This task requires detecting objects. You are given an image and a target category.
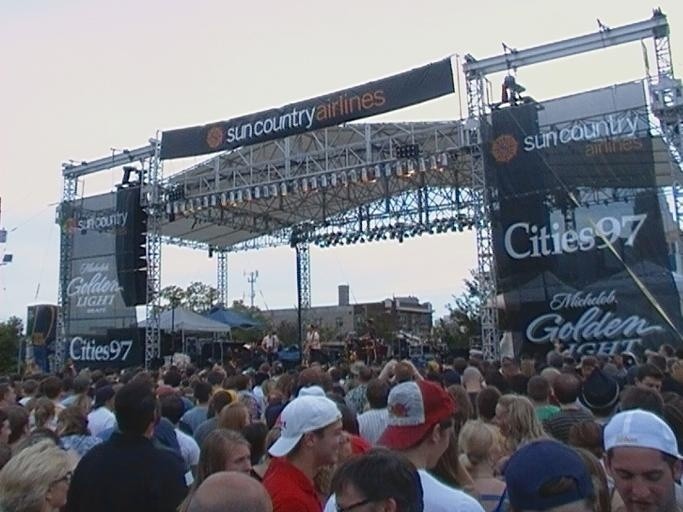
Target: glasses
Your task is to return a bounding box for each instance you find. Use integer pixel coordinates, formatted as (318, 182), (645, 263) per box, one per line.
(52, 471), (72, 485)
(335, 499), (374, 512)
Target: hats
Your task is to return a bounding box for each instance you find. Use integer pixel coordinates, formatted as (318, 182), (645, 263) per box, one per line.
(604, 409), (683, 461)
(376, 381), (456, 450)
(505, 440), (594, 511)
(93, 385), (114, 408)
(268, 395), (342, 458)
(579, 373), (621, 414)
(73, 375), (91, 387)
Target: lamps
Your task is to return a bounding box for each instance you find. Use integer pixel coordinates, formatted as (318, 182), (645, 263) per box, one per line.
(351, 170), (357, 183)
(430, 155), (437, 170)
(375, 164), (383, 178)
(418, 158), (425, 172)
(210, 195), (216, 207)
(396, 161), (403, 176)
(302, 178), (307, 193)
(362, 168), (367, 180)
(174, 201), (179, 213)
(203, 197), (209, 209)
(237, 190), (242, 200)
(441, 153), (448, 166)
(255, 187), (260, 198)
(384, 163), (391, 176)
(312, 176), (317, 189)
(281, 183), (287, 196)
(246, 188), (251, 200)
(195, 198), (201, 211)
(230, 192), (234, 202)
(342, 171), (347, 185)
(408, 159), (416, 173)
(270, 184), (277, 196)
(221, 194), (226, 203)
(183, 199), (195, 219)
(263, 186), (268, 197)
(292, 181), (298, 194)
(307, 214), (475, 248)
(321, 175), (327, 188)
(167, 204), (171, 213)
(331, 173), (337, 188)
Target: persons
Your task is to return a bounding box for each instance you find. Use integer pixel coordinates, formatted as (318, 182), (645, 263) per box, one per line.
(2, 325), (682, 512)
